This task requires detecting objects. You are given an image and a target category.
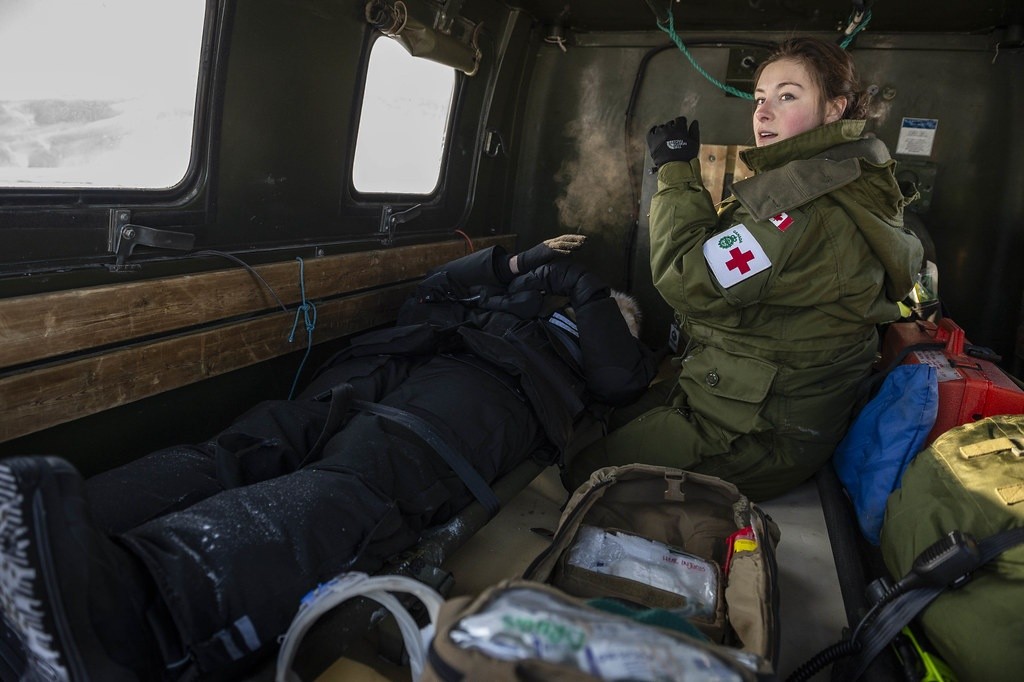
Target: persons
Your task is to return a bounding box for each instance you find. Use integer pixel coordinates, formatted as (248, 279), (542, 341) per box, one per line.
(558, 36), (925, 506)
(0, 233), (644, 682)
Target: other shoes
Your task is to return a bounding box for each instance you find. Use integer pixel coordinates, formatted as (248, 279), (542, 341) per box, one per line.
(1, 456), (153, 682)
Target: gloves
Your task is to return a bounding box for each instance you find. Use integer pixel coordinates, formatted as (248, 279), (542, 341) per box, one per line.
(516, 234), (587, 273)
(509, 263), (611, 306)
(646, 116), (700, 166)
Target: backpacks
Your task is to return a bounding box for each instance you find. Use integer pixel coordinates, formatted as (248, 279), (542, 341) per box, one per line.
(427, 461), (781, 682)
